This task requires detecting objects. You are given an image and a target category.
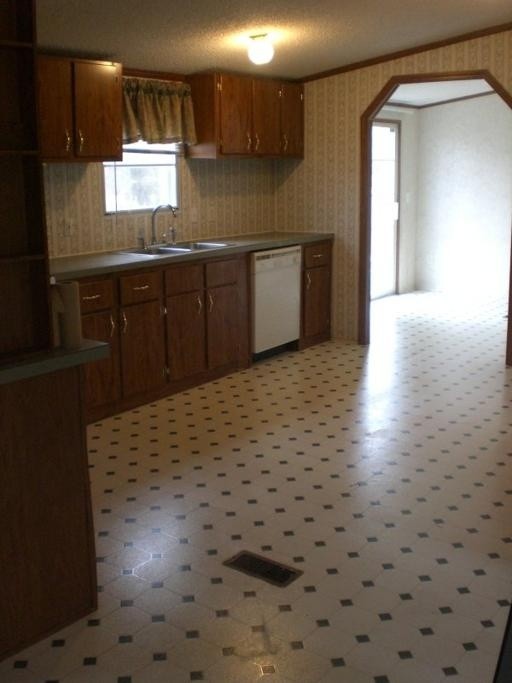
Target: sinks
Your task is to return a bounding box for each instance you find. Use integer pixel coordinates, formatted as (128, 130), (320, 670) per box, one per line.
(162, 242), (227, 250)
(126, 248), (186, 256)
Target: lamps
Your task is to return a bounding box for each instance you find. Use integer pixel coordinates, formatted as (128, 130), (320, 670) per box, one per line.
(247, 34), (274, 64)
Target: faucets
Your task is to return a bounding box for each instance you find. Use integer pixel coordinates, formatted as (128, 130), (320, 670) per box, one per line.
(151, 203), (176, 244)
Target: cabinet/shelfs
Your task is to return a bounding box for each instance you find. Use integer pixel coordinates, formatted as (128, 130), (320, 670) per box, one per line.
(185, 72), (281, 160)
(33, 53), (123, 161)
(72, 256), (169, 424)
(1, 1), (111, 661)
(164, 244), (250, 395)
(281, 81), (304, 158)
(287, 234), (331, 350)
